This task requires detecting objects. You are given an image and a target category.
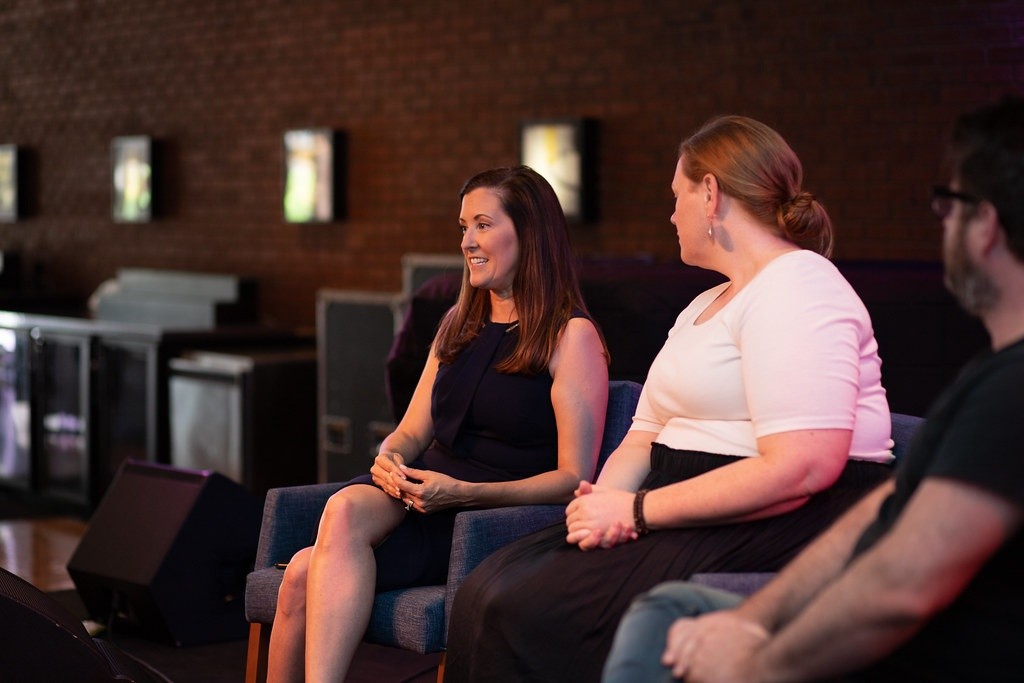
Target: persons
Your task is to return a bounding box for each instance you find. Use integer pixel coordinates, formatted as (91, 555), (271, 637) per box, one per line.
(663, 110), (1024, 683)
(266, 168), (608, 683)
(444, 118), (894, 682)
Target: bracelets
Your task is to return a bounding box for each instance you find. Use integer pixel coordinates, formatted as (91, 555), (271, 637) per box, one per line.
(633, 490), (648, 536)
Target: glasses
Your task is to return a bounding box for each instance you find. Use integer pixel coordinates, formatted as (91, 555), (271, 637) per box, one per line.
(927, 185), (980, 216)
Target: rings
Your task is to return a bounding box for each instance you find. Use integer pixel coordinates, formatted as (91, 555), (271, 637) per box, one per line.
(405, 500), (413, 511)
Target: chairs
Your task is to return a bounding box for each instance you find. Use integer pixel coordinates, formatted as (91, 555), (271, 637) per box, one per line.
(685, 410), (922, 597)
(244, 380), (644, 683)
